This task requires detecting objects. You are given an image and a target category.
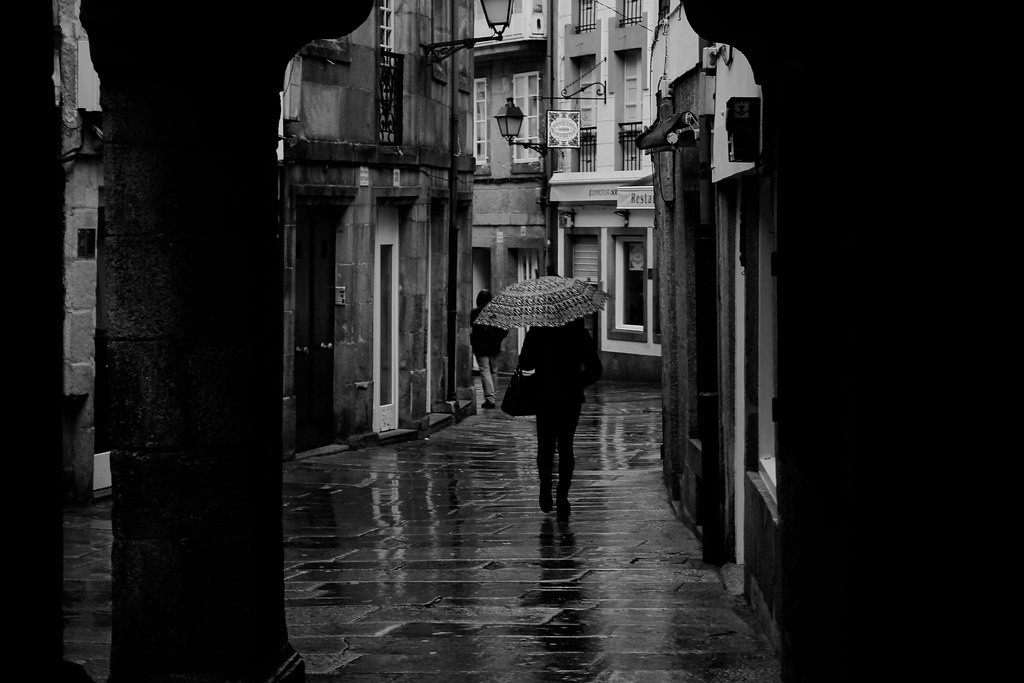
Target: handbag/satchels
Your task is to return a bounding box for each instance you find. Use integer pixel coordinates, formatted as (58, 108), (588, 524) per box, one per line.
(502, 365), (547, 417)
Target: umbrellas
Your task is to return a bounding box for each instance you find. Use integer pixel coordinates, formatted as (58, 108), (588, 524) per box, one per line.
(473, 276), (611, 331)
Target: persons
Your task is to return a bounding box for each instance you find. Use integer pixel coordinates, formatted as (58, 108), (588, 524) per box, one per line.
(518, 317), (604, 513)
(470, 289), (509, 409)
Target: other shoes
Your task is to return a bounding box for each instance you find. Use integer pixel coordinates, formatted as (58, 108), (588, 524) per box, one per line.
(482, 401), (496, 409)
(539, 475), (553, 513)
(556, 487), (571, 511)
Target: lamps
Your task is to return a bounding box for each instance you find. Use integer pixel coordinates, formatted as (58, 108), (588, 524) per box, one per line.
(419, 0), (514, 74)
(494, 96), (548, 156)
